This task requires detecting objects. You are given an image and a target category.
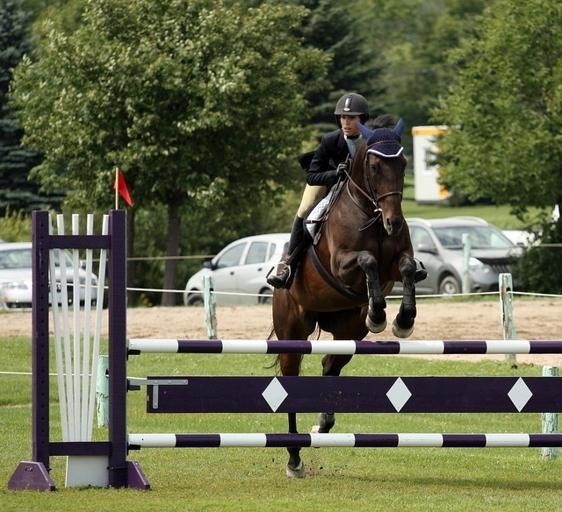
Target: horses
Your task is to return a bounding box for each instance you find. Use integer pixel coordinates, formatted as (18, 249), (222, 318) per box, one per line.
(262, 113), (418, 478)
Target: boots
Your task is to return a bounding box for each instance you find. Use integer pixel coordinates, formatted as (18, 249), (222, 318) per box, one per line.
(266, 255), (297, 290)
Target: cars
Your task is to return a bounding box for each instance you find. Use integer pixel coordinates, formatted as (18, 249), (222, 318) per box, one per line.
(184, 233), (290, 306)
(389, 219), (525, 300)
(1, 241), (101, 312)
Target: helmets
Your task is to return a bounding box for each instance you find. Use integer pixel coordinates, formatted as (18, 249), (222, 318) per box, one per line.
(334, 92), (370, 132)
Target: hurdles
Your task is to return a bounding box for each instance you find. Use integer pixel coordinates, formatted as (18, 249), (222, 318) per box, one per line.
(9, 210), (562, 494)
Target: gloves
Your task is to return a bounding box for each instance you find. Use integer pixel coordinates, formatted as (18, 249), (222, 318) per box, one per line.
(335, 162), (348, 177)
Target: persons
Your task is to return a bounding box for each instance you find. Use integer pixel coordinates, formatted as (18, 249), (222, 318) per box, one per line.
(267, 92), (428, 290)
